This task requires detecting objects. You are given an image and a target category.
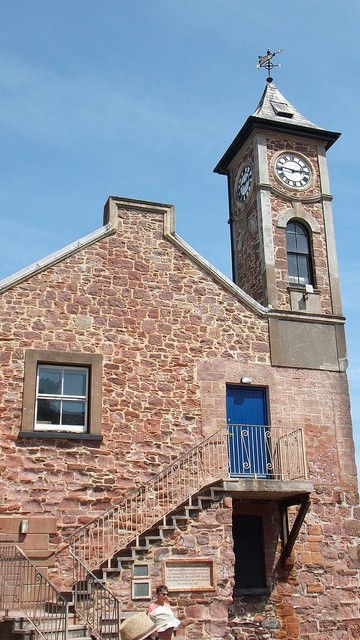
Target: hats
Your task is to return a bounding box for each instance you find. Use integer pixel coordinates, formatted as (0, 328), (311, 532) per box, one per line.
(150, 605), (180, 632)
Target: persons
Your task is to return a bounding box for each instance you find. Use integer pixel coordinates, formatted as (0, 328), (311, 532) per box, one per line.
(148, 586), (171, 611)
(149, 606), (181, 640)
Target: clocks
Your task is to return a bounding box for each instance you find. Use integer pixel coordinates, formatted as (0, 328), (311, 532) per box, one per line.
(234, 160), (254, 208)
(272, 150), (317, 192)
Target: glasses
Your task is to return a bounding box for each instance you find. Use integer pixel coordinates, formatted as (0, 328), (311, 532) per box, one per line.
(158, 593), (168, 595)
